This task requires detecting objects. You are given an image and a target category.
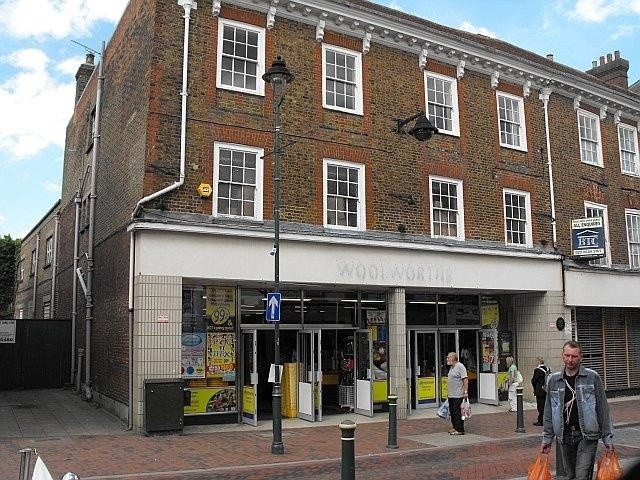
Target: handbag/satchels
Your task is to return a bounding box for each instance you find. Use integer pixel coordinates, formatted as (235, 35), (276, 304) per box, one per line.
(517, 370), (524, 384)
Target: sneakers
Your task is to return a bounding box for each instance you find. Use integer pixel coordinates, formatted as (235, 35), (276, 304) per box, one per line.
(448, 429), (456, 433)
(450, 431), (466, 436)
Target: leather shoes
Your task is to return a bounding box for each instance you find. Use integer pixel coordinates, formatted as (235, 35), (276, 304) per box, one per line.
(533, 422), (542, 426)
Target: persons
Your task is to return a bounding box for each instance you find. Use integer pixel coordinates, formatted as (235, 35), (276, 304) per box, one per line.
(445, 351), (469, 436)
(540, 341), (613, 480)
(506, 356), (523, 412)
(531, 355), (550, 425)
(373, 345), (385, 368)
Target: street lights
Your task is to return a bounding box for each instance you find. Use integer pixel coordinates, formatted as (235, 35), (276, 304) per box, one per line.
(259, 52), (296, 455)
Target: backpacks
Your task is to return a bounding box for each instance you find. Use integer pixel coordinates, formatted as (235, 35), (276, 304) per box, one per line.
(538, 366), (552, 390)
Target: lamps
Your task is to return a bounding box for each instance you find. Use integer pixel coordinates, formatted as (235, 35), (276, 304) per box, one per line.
(393, 109), (440, 141)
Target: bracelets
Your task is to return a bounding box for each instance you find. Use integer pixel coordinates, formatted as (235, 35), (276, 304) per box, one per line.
(464, 391), (468, 394)
